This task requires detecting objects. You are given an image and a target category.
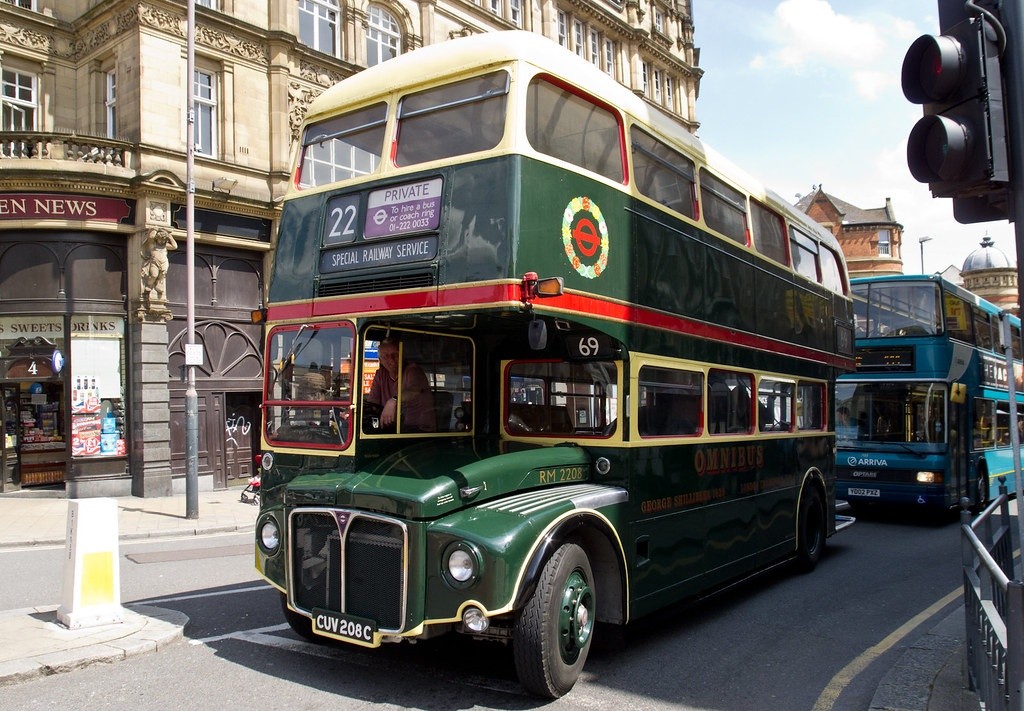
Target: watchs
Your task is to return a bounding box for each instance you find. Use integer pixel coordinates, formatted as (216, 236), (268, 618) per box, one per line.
(391, 395), (398, 402)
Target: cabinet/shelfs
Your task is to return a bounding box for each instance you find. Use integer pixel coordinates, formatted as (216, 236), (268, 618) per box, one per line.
(0, 382), (64, 488)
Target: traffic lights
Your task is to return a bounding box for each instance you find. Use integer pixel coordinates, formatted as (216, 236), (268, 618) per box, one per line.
(900, 12), (1011, 208)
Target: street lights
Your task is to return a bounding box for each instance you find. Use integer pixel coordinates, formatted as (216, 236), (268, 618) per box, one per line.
(919, 235), (932, 272)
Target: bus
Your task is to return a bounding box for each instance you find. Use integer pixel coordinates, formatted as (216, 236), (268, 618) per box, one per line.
(835, 274), (1024, 528)
(246, 29), (856, 698)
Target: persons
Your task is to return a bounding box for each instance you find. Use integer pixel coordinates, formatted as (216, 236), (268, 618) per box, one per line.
(362, 342), (444, 433)
(836, 406), (869, 442)
(145, 224), (179, 296)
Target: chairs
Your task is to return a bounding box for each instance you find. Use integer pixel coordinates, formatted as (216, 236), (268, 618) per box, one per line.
(430, 334), (1023, 448)
